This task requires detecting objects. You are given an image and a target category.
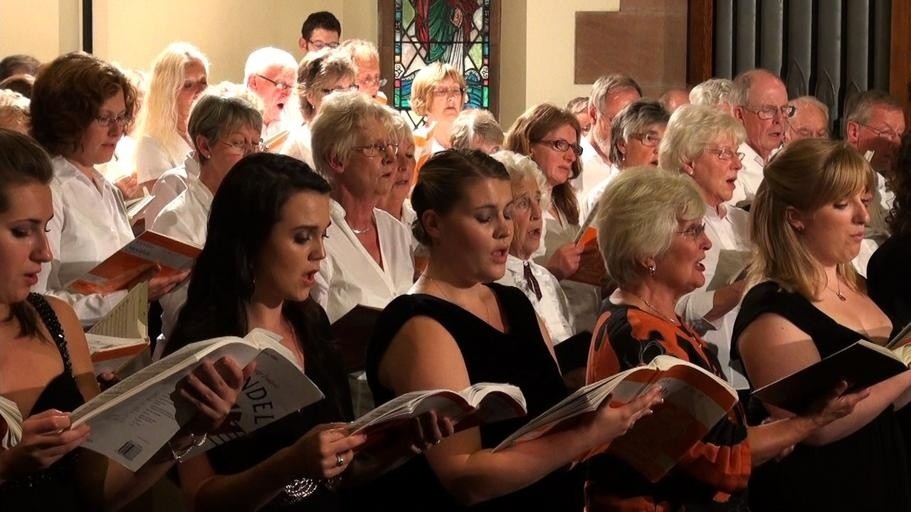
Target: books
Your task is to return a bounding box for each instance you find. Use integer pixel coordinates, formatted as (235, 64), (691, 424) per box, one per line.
(0, 396), (25, 451)
(885, 313), (911, 350)
(568, 200), (618, 290)
(491, 353), (739, 486)
(749, 321), (911, 436)
(376, 90), (388, 104)
(84, 279), (151, 380)
(727, 250), (764, 286)
(330, 303), (384, 371)
(336, 382), (527, 450)
(262, 130), (290, 153)
(125, 195), (156, 220)
(862, 149), (876, 167)
(412, 120), (437, 186)
(63, 229), (206, 295)
(55, 327), (325, 474)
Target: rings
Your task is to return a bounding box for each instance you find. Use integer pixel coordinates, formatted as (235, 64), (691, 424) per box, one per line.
(430, 438), (442, 446)
(337, 453), (343, 467)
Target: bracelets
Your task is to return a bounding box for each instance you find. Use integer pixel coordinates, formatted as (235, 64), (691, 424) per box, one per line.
(189, 428), (208, 448)
(167, 439), (194, 465)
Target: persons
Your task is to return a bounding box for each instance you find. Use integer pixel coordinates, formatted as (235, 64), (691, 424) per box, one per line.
(281, 48), (360, 174)
(839, 90), (907, 278)
(0, 53), (42, 137)
(609, 99), (669, 172)
(727, 70), (789, 208)
(365, 147), (664, 511)
(582, 163), (871, 512)
(112, 41), (210, 185)
(567, 97), (593, 137)
(18, 50), (193, 380)
(731, 140), (911, 512)
(448, 108), (506, 157)
(243, 47), (307, 155)
(298, 11), (342, 58)
(377, 104), (429, 263)
(688, 77), (736, 116)
(489, 150), (577, 348)
(503, 103), (607, 336)
(656, 104), (768, 390)
(149, 82), (265, 251)
(569, 72), (645, 230)
(787, 95), (830, 146)
(658, 87), (691, 115)
(408, 61), (469, 200)
(866, 132), (911, 341)
(0, 127), (257, 512)
(341, 38), (388, 103)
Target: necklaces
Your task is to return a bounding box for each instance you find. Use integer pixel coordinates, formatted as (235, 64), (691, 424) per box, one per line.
(818, 275), (847, 303)
(346, 206), (375, 235)
(621, 286), (696, 341)
(427, 260), (490, 326)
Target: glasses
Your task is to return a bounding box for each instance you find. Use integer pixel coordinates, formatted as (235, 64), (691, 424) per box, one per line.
(220, 139), (263, 153)
(534, 139), (583, 156)
(738, 103), (796, 119)
(94, 113), (128, 126)
(858, 122), (904, 143)
(357, 76), (388, 87)
(305, 39), (339, 50)
(352, 143), (400, 157)
(256, 74), (294, 92)
(674, 223), (707, 240)
(706, 148), (745, 161)
(630, 131), (662, 146)
(319, 84), (359, 94)
